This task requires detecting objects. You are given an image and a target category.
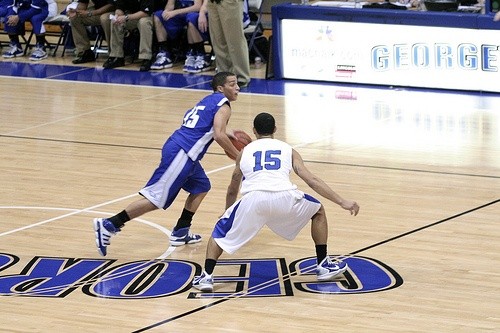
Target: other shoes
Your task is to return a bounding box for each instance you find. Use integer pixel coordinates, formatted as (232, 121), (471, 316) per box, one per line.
(71, 49), (97, 64)
(139, 59), (152, 72)
(237, 80), (248, 89)
(103, 56), (125, 69)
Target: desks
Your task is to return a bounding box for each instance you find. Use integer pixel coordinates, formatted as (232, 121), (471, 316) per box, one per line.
(272, 4), (500, 97)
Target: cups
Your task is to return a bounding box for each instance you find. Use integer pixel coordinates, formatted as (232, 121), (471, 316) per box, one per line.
(254, 57), (261, 69)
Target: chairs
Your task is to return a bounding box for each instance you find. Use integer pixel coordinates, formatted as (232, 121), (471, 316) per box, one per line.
(0, 0), (266, 65)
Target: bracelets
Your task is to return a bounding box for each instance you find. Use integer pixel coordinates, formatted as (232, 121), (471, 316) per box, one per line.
(124, 14), (128, 22)
(88, 10), (92, 17)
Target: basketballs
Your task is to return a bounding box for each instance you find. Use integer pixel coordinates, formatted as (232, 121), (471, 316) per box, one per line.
(224, 131), (252, 160)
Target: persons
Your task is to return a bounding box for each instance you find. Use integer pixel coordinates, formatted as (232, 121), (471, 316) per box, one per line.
(0, 0), (264, 90)
(192, 113), (360, 291)
(94, 72), (240, 257)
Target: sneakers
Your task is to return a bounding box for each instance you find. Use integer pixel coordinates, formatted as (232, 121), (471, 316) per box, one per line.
(2, 43), (24, 58)
(315, 255), (348, 282)
(191, 268), (214, 292)
(183, 51), (212, 74)
(29, 42), (48, 61)
(93, 217), (122, 257)
(150, 51), (174, 70)
(169, 226), (202, 246)
